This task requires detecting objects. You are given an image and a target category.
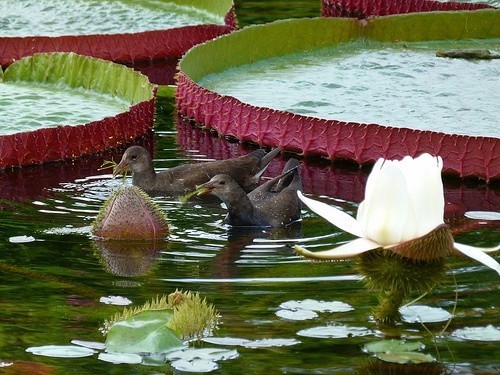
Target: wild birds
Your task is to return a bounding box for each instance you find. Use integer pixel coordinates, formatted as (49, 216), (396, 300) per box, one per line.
(196, 157), (304, 228)
(111, 144), (282, 192)
